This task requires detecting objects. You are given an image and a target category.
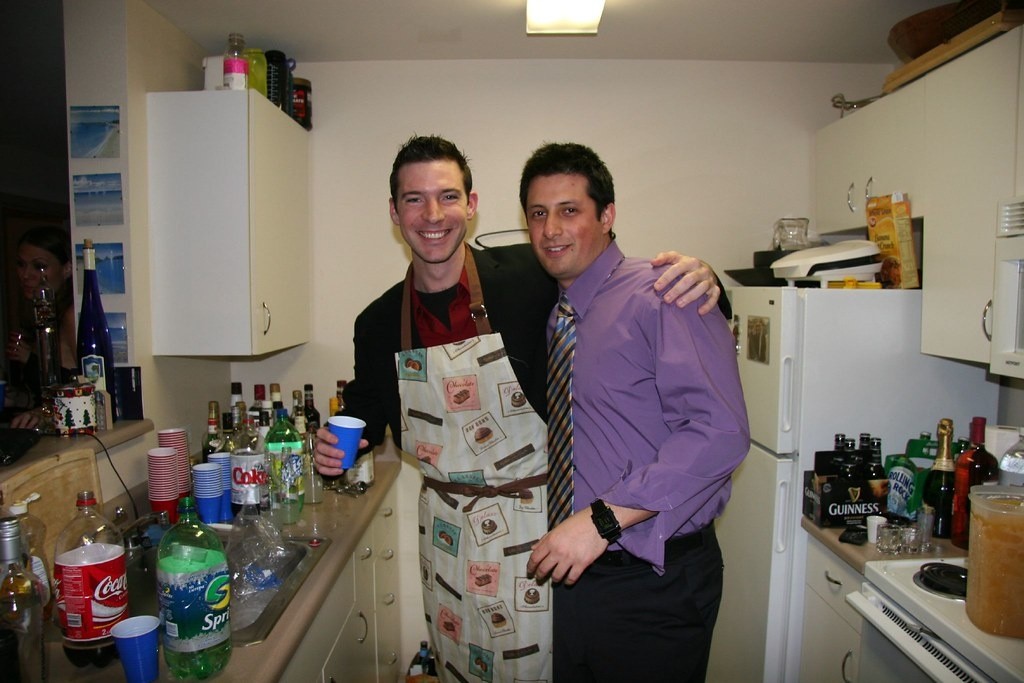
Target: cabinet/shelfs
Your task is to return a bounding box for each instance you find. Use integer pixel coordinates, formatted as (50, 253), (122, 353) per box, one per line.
(275, 469), (407, 683)
(797, 530), (875, 682)
(812, 89), (924, 235)
(919, 24), (1024, 381)
(142, 92), (316, 359)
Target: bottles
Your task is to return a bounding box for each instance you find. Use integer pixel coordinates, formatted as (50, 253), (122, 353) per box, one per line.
(0, 490), (128, 683)
(223, 33), (313, 131)
(773, 218), (812, 251)
(829, 434), (888, 495)
(202, 420), (222, 464)
(920, 417), (1024, 551)
(214, 413), (241, 452)
(32, 239), (116, 434)
(208, 401), (219, 419)
(155, 496), (232, 683)
(232, 380), (375, 527)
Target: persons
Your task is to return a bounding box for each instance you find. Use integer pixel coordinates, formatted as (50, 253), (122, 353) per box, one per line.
(6, 225), (79, 434)
(312, 132), (734, 683)
(517, 138), (751, 683)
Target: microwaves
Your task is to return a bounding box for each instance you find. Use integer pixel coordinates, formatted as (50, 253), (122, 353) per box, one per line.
(990, 195), (1024, 381)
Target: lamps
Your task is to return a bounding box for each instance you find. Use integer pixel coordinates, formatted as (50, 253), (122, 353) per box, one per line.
(525, 0), (607, 35)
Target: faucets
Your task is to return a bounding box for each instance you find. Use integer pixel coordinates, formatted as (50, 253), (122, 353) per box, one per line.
(76, 506), (170, 546)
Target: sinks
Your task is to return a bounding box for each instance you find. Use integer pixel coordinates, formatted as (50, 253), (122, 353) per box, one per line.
(116, 510), (333, 648)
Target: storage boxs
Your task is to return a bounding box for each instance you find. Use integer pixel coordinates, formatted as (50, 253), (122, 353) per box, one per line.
(869, 189), (919, 292)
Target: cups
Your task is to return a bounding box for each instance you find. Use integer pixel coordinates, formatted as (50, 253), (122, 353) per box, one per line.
(109, 613), (161, 683)
(147, 428), (231, 524)
(51, 376), (97, 437)
(0, 380), (6, 412)
(327, 416), (366, 469)
(866, 507), (919, 554)
(917, 506), (936, 552)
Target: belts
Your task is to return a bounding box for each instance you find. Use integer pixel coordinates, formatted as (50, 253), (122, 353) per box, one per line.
(594, 522), (716, 567)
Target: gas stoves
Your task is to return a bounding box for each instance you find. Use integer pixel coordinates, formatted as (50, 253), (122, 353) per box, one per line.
(864, 557), (1024, 683)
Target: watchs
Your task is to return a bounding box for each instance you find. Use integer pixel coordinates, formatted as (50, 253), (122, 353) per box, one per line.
(588, 498), (623, 544)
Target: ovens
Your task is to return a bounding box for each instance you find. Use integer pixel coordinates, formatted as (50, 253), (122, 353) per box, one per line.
(845, 582), (999, 683)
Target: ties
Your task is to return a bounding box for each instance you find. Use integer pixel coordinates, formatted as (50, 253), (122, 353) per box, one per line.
(547, 258), (624, 531)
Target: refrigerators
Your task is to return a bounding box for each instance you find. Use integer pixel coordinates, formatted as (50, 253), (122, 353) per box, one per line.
(705, 286), (1000, 683)
